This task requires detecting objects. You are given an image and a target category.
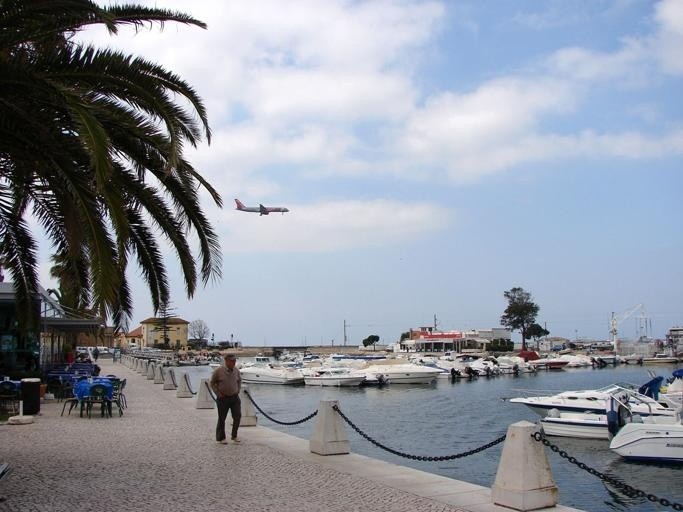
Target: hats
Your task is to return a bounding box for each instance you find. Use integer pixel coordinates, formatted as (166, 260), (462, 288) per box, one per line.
(224, 354), (239, 360)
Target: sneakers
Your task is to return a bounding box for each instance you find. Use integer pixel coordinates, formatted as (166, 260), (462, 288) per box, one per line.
(231, 436), (241, 442)
(220, 439), (228, 444)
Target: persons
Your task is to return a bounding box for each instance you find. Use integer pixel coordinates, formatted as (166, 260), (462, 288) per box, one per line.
(208, 350), (243, 445)
(91, 345), (98, 361)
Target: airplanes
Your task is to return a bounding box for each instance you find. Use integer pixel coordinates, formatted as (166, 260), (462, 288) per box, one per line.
(233, 199), (289, 216)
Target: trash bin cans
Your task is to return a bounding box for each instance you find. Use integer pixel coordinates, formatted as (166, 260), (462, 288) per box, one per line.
(20, 378), (41, 415)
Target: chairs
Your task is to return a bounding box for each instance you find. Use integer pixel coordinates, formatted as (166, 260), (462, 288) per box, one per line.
(0, 359), (127, 419)
